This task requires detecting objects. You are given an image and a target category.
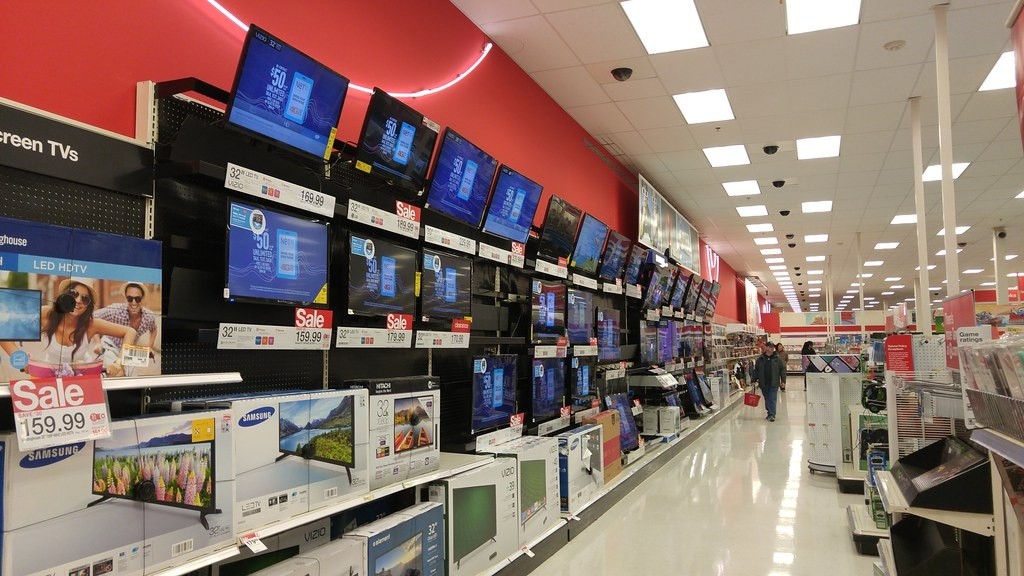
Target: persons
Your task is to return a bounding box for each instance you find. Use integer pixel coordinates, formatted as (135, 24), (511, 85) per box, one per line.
(0, 277), (136, 378)
(90, 282), (157, 376)
(802, 341), (816, 391)
(773, 343), (789, 391)
(751, 341), (786, 421)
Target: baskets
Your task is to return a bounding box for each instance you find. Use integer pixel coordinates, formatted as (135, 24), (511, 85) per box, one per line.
(745, 393), (761, 407)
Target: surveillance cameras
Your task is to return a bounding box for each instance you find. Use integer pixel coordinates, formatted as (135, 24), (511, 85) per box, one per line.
(773, 180), (785, 188)
(611, 68), (633, 82)
(997, 230), (1007, 238)
(780, 211), (790, 216)
(786, 235), (807, 310)
(763, 146), (778, 155)
(958, 243), (967, 247)
(935, 293), (939, 295)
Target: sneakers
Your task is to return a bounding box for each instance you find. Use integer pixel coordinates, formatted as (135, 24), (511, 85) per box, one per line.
(768, 416), (775, 421)
(766, 412), (768, 419)
(780, 386), (785, 392)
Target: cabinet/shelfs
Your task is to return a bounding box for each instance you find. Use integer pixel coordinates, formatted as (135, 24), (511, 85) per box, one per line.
(872, 469), (994, 576)
(781, 343), (830, 373)
(153, 451), (511, 576)
(725, 323), (769, 393)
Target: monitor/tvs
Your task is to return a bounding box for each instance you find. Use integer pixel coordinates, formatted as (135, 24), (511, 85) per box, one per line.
(226, 21), (722, 453)
(393, 395), (434, 454)
(452, 484), (496, 571)
(374, 530), (425, 576)
(520, 459), (547, 532)
(88, 417), (222, 530)
(276, 395), (355, 485)
(0, 287), (42, 348)
(581, 428), (600, 484)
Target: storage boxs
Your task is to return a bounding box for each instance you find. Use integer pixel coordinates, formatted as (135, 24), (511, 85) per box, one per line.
(0, 368), (729, 576)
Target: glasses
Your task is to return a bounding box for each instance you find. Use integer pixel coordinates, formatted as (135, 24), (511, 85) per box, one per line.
(642, 188), (649, 198)
(127, 295), (141, 303)
(652, 197), (659, 204)
(69, 288), (90, 305)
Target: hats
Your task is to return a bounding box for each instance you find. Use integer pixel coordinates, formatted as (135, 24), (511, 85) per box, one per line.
(120, 282), (149, 301)
(58, 277), (98, 305)
(766, 342), (777, 347)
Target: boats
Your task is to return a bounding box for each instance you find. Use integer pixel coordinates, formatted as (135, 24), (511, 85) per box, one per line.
(418, 427), (430, 447)
(395, 426), (415, 452)
(394, 430), (405, 451)
